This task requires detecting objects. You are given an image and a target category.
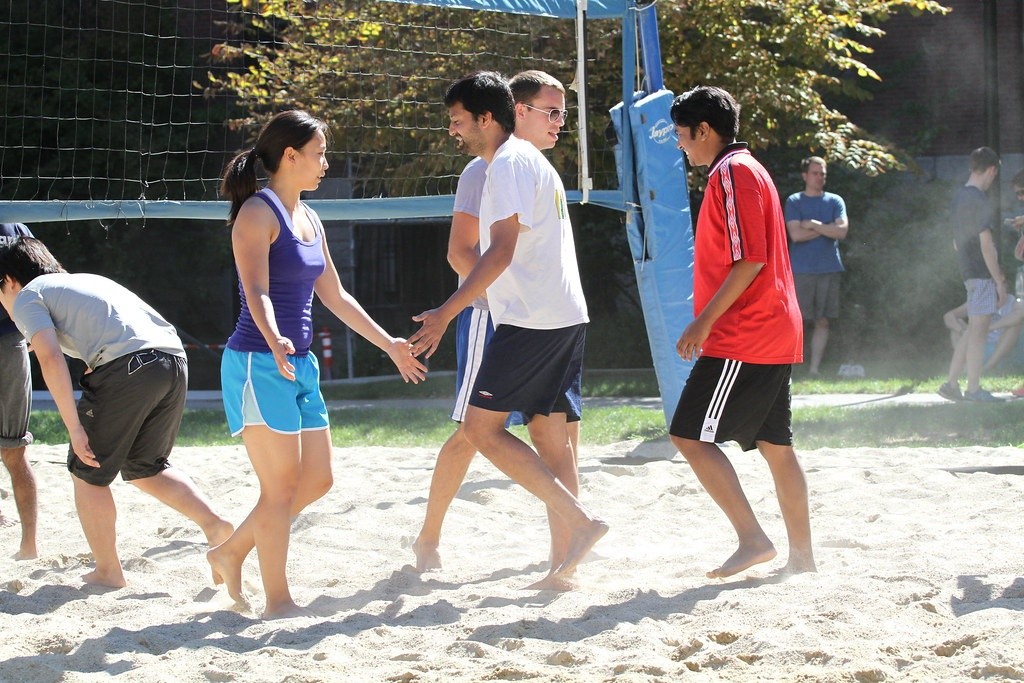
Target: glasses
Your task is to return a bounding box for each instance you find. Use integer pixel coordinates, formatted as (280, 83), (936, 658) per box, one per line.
(519, 102), (568, 124)
(1015, 189), (1024, 196)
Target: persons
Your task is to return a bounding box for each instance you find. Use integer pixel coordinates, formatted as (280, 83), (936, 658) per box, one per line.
(415, 71), (586, 570)
(207, 110), (427, 622)
(937, 147), (1024, 402)
(0, 223), (38, 561)
(785, 156), (848, 380)
(669, 86), (819, 577)
(0, 237), (234, 587)
(404, 71), (608, 580)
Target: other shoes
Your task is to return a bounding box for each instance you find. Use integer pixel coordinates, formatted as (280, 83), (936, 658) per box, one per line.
(1011, 381), (1024, 396)
(935, 381), (964, 402)
(964, 388), (1005, 403)
(807, 370), (824, 380)
